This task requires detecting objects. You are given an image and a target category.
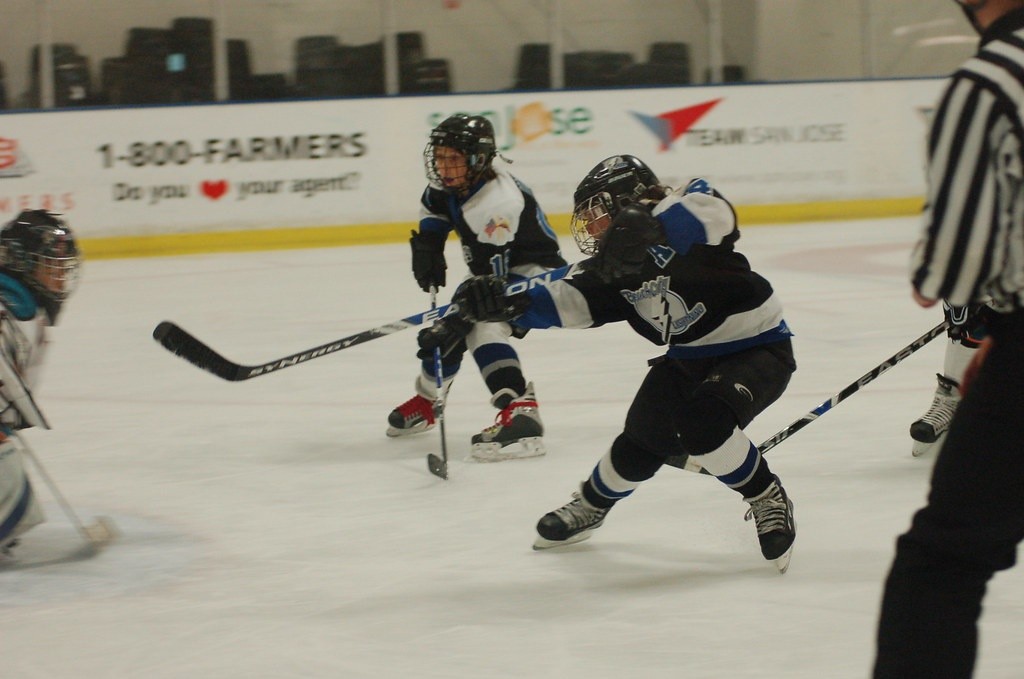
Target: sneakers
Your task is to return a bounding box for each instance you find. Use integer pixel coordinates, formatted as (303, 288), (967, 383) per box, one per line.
(743, 474), (796, 574)
(385, 376), (453, 437)
(470, 381), (547, 462)
(532, 480), (622, 550)
(909, 373), (962, 458)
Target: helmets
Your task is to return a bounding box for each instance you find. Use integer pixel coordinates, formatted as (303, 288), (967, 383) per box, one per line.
(423, 113), (496, 198)
(569, 154), (658, 255)
(0, 208), (79, 324)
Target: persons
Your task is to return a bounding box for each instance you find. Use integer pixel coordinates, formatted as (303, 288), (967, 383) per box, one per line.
(0, 209), (109, 573)
(387, 112), (567, 462)
(871, 0), (1024, 679)
(453, 153), (797, 573)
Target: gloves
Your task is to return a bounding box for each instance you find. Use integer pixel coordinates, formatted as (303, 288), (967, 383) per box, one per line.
(594, 203), (668, 284)
(450, 274), (524, 323)
(410, 229), (447, 292)
(943, 298), (993, 341)
(416, 313), (474, 361)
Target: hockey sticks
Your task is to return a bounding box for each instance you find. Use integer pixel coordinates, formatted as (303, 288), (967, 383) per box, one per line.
(152, 258), (595, 383)
(426, 277), (449, 481)
(650, 322), (949, 477)
(9, 427), (122, 547)
